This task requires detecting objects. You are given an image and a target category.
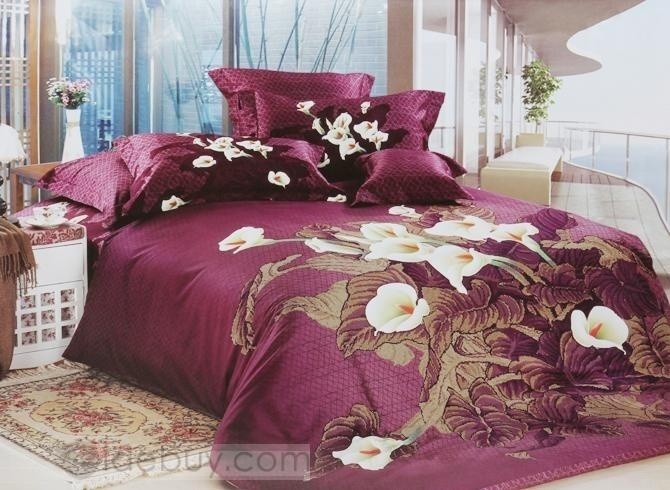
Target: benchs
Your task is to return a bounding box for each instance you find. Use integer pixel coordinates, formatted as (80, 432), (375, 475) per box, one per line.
(479, 144), (565, 207)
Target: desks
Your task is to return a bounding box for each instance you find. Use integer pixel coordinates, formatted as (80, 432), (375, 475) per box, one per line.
(9, 160), (61, 212)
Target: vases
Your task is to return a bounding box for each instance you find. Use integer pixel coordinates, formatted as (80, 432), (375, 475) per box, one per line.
(61, 109), (85, 167)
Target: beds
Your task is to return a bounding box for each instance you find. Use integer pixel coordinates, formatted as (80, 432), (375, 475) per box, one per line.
(7, 186), (670, 490)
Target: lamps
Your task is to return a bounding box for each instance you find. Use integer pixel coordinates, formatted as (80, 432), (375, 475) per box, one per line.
(0, 123), (27, 217)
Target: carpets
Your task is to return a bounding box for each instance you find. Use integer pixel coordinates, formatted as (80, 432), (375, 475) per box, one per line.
(2, 364), (220, 479)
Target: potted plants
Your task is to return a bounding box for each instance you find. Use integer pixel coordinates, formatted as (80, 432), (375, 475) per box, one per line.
(514, 59), (566, 146)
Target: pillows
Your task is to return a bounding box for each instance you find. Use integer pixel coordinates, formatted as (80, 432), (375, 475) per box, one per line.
(207, 68), (376, 139)
(352, 149), (476, 212)
(116, 129), (342, 220)
(35, 149), (133, 231)
(234, 83), (445, 183)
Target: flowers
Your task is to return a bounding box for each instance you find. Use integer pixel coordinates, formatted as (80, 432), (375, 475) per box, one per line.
(46, 75), (96, 110)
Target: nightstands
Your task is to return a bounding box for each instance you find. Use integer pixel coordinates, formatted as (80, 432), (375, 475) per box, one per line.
(9, 220), (88, 373)
